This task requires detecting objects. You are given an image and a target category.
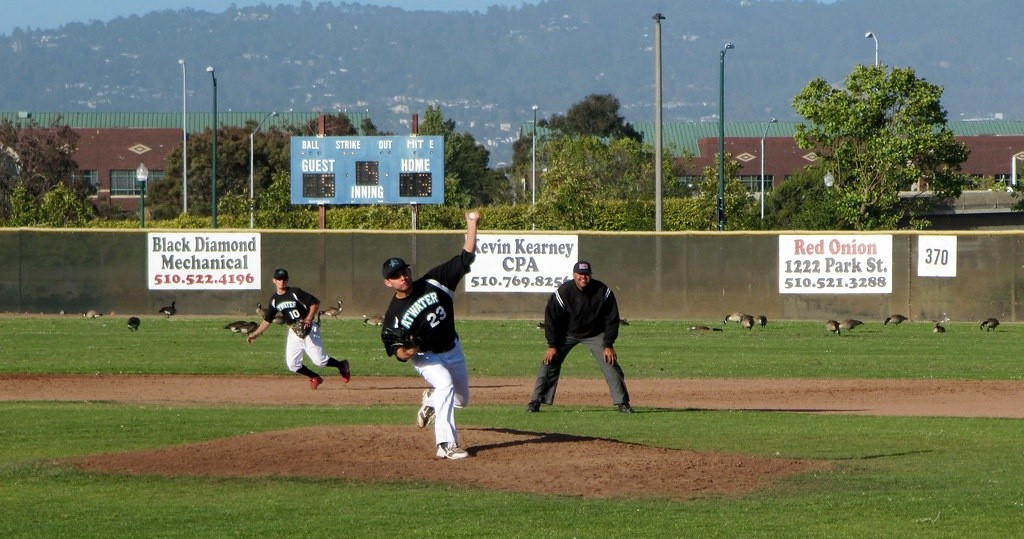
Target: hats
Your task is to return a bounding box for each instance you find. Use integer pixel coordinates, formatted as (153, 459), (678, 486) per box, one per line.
(382, 257), (410, 279)
(273, 269), (288, 279)
(573, 261), (592, 274)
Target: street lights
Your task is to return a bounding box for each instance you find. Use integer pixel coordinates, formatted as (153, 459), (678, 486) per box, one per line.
(865, 31), (880, 67)
(532, 105), (539, 230)
(651, 12), (666, 232)
(206, 66), (219, 228)
(136, 161), (148, 228)
(177, 59), (187, 214)
(249, 111), (280, 228)
(760, 116), (779, 219)
(718, 41), (735, 230)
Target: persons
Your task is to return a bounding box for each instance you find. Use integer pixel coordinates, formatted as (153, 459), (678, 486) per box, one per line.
(247, 268), (350, 390)
(529, 260), (633, 413)
(380, 206), (481, 459)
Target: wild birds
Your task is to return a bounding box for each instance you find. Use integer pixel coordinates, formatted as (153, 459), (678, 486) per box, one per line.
(323, 300), (343, 320)
(272, 317), (285, 326)
(537, 323), (545, 331)
(314, 311), (323, 326)
(256, 303), (268, 318)
(159, 301), (176, 318)
(127, 317), (140, 333)
(686, 325), (723, 332)
(620, 318), (629, 326)
(224, 321), (260, 336)
(362, 315), (385, 327)
(824, 313), (1001, 335)
(723, 312), (767, 330)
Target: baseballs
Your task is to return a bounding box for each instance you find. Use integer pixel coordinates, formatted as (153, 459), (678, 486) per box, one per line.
(469, 212), (476, 219)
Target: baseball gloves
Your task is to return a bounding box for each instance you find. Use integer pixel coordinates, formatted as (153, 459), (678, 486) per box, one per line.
(291, 321), (312, 338)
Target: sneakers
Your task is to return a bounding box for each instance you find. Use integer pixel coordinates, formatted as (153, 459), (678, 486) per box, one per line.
(416, 388), (436, 429)
(527, 400), (540, 412)
(437, 442), (469, 459)
(618, 403), (633, 414)
(338, 360), (350, 383)
(309, 375), (323, 390)
